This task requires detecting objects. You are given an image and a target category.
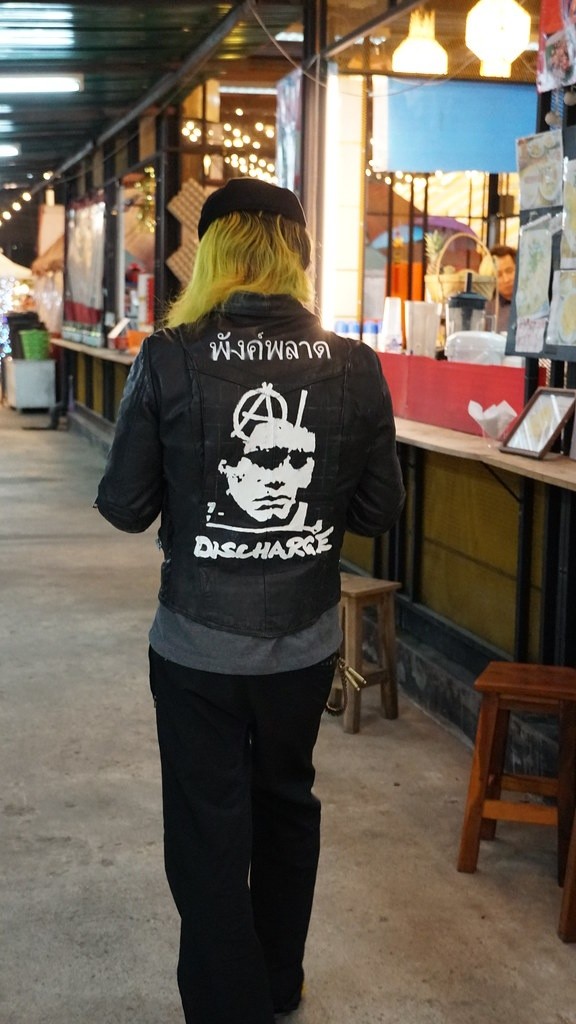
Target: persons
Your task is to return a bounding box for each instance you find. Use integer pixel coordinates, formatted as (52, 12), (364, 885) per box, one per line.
(485, 246), (516, 334)
(91, 178), (406, 1024)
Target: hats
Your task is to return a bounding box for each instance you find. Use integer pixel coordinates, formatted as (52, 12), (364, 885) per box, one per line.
(197, 178), (307, 241)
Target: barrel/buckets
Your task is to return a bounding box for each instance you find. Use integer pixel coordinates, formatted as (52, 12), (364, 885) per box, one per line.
(19, 330), (48, 361)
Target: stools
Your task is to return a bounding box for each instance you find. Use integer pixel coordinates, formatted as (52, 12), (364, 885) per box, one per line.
(327, 571), (403, 733)
(456, 661), (576, 944)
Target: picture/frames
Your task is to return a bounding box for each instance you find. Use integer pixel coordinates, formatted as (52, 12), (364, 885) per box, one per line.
(500, 385), (576, 460)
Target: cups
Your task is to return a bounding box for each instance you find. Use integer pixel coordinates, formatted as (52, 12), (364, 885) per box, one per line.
(333, 296), (443, 358)
